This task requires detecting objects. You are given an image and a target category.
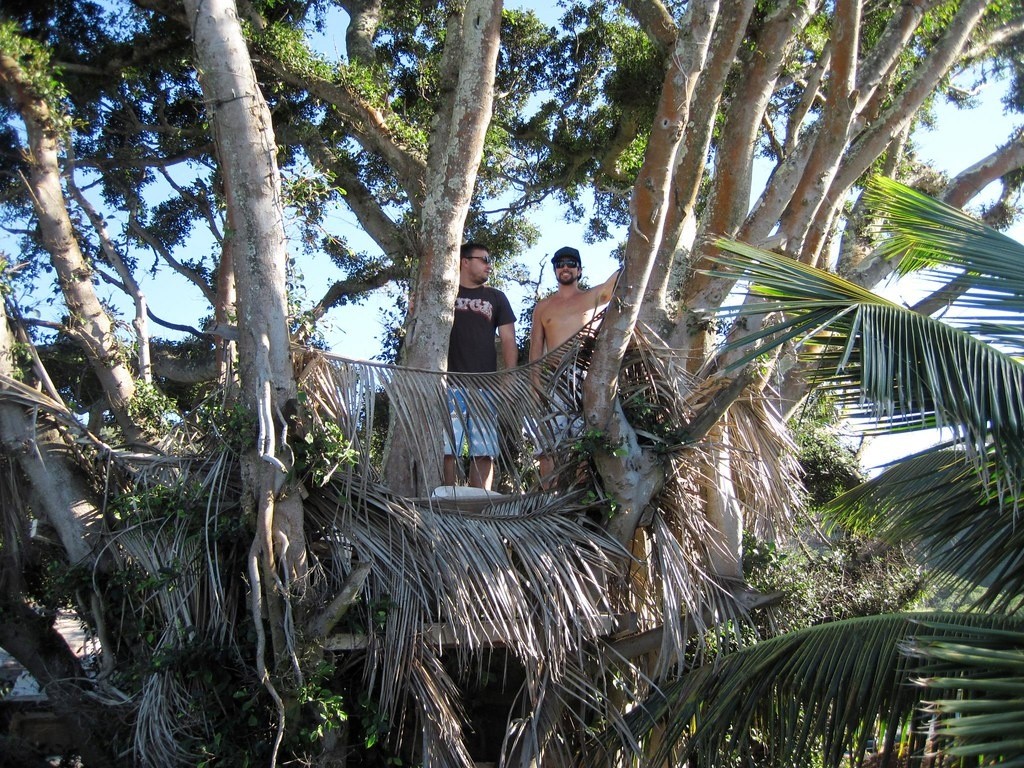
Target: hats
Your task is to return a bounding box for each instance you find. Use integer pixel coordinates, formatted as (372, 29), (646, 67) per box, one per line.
(551, 246), (581, 268)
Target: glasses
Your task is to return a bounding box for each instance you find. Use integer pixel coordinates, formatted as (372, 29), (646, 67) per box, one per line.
(465, 256), (491, 264)
(553, 259), (580, 268)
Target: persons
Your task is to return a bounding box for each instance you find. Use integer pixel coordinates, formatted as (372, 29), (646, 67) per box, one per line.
(406, 244), (518, 492)
(528, 246), (621, 491)
